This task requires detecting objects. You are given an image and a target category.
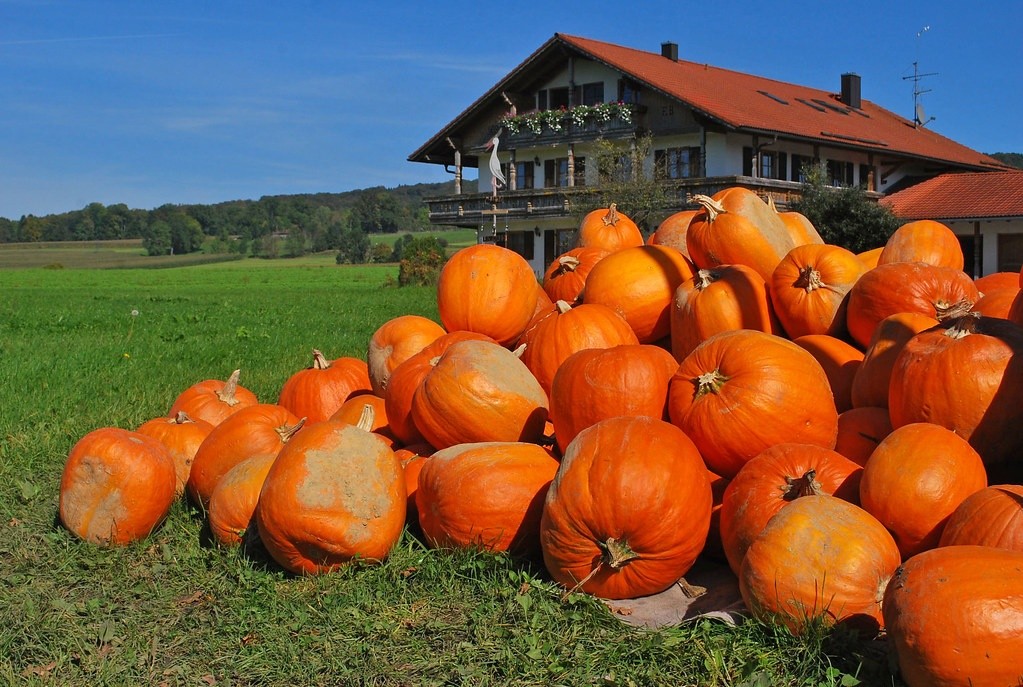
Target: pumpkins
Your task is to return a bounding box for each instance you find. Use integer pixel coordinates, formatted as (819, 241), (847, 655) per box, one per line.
(60, 188), (1023, 687)
